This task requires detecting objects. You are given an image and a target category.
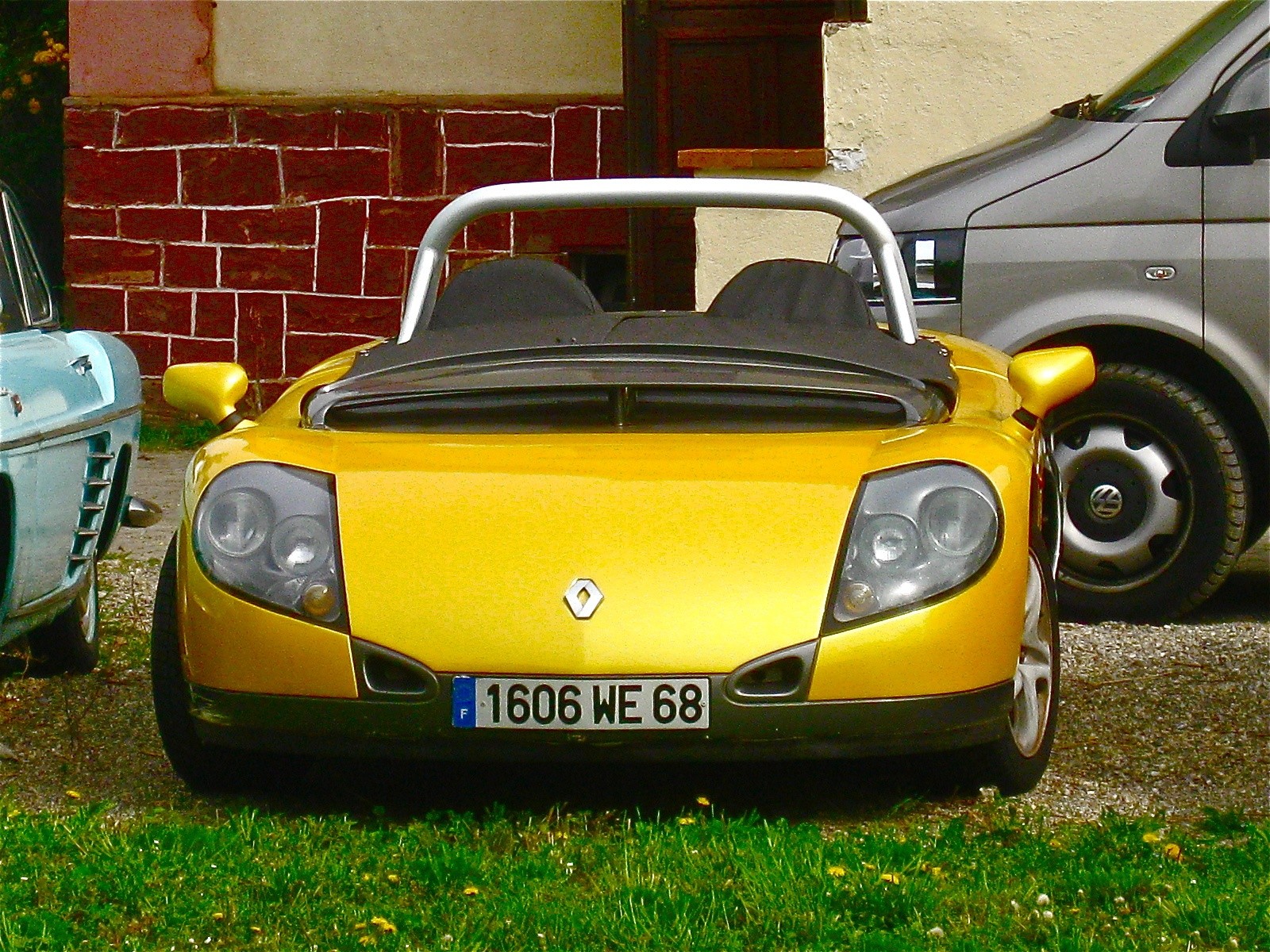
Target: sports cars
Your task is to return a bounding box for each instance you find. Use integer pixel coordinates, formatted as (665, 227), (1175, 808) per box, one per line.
(145, 171), (1102, 801)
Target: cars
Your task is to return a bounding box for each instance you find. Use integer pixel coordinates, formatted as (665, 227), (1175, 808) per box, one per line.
(0, 183), (141, 682)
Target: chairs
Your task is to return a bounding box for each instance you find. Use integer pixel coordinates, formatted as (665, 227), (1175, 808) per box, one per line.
(424, 256), (601, 329)
(705, 258), (879, 330)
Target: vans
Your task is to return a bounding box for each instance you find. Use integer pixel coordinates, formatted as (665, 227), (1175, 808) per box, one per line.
(830, 1), (1270, 628)
